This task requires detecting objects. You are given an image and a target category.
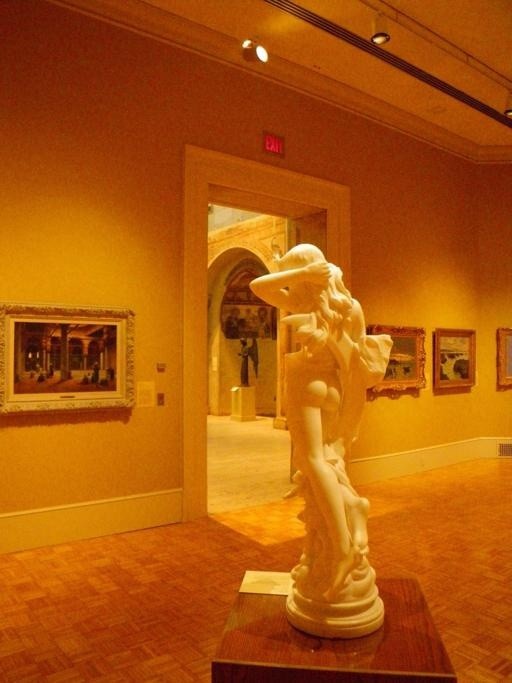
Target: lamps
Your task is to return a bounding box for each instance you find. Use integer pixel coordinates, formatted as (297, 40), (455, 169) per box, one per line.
(237, 36), (270, 68)
(370, 7), (392, 45)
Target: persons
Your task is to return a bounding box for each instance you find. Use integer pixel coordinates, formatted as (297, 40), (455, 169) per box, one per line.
(30, 360), (114, 386)
(225, 307), (271, 338)
(250, 242), (394, 595)
(238, 337), (249, 384)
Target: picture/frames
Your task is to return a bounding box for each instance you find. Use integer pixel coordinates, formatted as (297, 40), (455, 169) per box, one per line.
(495, 328), (511, 393)
(0, 304), (140, 420)
(367, 323), (478, 394)
